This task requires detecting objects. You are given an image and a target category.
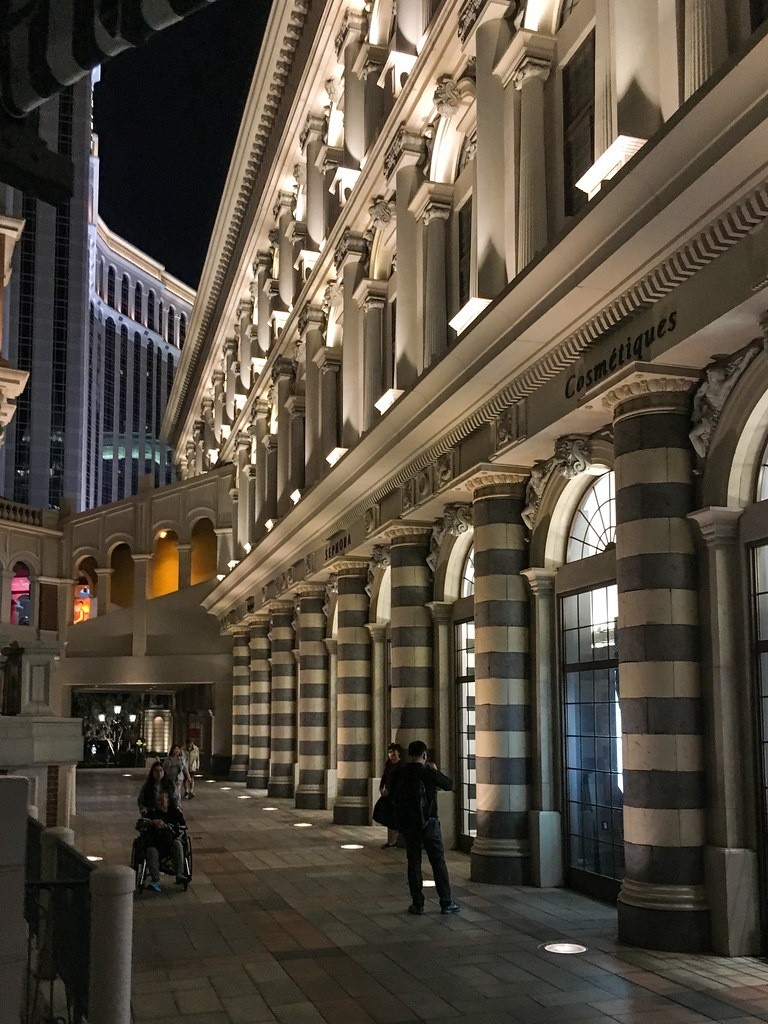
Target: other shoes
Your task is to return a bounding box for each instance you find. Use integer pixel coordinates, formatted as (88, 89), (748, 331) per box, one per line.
(184, 792), (189, 799)
(147, 882), (162, 892)
(407, 905), (425, 915)
(187, 792), (195, 800)
(383, 842), (398, 849)
(175, 876), (193, 885)
(440, 900), (462, 914)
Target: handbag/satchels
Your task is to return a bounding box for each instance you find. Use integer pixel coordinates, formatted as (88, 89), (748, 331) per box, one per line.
(372, 794), (393, 827)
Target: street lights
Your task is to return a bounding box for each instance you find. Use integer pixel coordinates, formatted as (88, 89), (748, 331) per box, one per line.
(98, 702), (137, 764)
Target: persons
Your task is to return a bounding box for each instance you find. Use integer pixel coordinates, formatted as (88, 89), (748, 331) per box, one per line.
(131, 761), (193, 893)
(163, 738), (199, 799)
(372, 741), (462, 915)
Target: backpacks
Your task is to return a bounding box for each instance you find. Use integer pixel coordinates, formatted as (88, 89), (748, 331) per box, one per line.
(392, 766), (431, 830)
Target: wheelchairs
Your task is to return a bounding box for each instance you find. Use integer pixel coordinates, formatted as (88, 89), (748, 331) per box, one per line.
(130, 832), (193, 894)
